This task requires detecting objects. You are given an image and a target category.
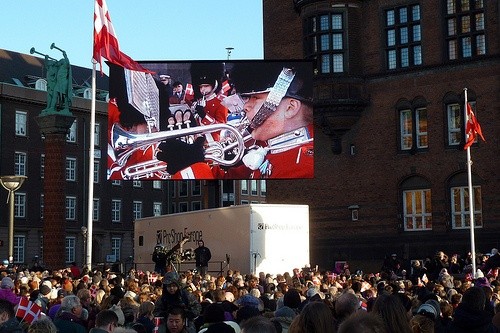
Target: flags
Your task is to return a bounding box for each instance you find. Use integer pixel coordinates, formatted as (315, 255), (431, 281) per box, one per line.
(16, 297), (34, 318)
(23, 303), (42, 323)
(221, 79), (231, 93)
(463, 102), (487, 151)
(90, 284), (99, 294)
(93, 0), (156, 78)
(465, 273), (472, 282)
(487, 270), (493, 276)
(149, 274), (158, 282)
(186, 83), (194, 95)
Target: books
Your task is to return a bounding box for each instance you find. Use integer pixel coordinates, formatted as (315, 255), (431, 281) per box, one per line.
(123, 66), (161, 132)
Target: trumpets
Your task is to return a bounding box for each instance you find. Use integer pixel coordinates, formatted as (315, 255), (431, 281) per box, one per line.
(110, 116), (258, 179)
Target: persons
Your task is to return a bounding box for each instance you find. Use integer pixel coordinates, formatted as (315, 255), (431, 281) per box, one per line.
(107, 60), (314, 179)
(43, 52), (71, 112)
(194, 240), (212, 275)
(152, 239), (167, 273)
(0, 248), (500, 333)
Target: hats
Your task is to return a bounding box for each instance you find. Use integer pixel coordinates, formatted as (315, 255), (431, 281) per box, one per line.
(482, 253), (491, 256)
(475, 269), (484, 280)
(124, 291), (136, 300)
(417, 304), (437, 320)
(490, 249), (498, 257)
(239, 60), (314, 102)
(173, 81), (181, 87)
(62, 279), (73, 291)
(1, 275), (14, 289)
(162, 272), (179, 284)
(306, 288), (318, 297)
(196, 71), (213, 84)
(39, 280), (52, 295)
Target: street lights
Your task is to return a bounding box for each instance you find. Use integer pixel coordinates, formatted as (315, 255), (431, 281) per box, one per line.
(0, 174), (29, 268)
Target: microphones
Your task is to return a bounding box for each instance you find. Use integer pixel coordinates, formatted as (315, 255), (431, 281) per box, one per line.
(226, 254), (230, 261)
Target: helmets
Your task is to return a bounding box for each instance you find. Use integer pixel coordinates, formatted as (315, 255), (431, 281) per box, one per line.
(233, 294), (264, 312)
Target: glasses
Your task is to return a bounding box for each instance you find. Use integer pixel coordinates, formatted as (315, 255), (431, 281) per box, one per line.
(174, 86), (181, 88)
